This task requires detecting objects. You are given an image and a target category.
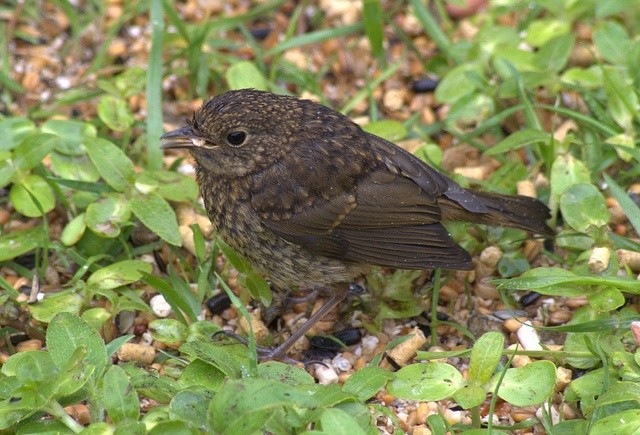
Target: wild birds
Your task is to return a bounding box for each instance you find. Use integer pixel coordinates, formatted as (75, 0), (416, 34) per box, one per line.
(158, 89), (557, 368)
